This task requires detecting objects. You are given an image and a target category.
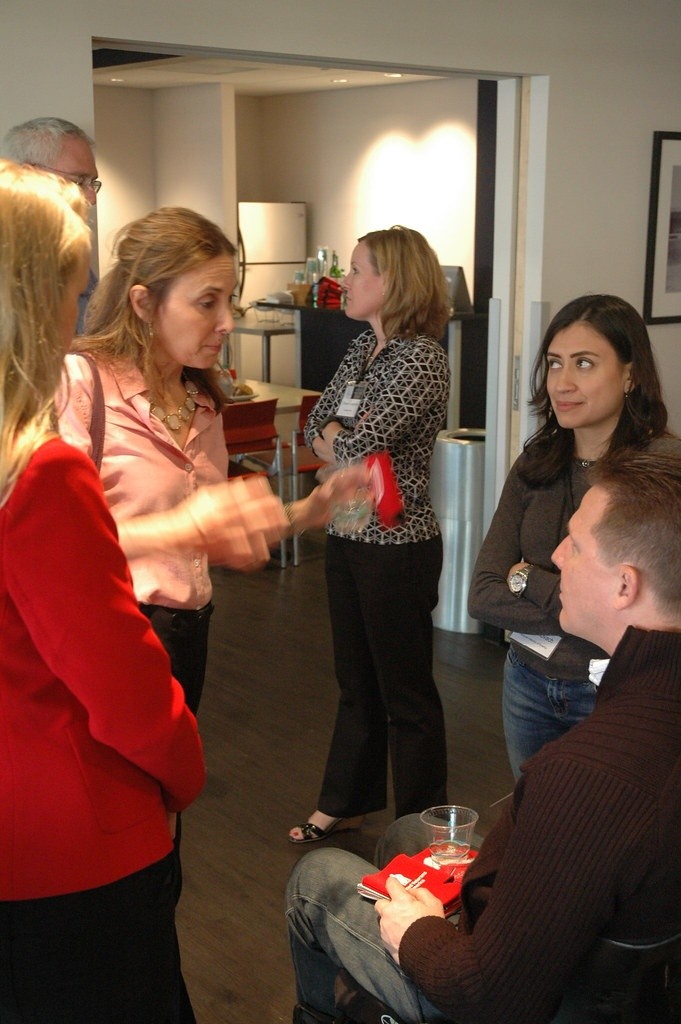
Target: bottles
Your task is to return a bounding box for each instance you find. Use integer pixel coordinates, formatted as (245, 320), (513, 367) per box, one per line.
(330, 250), (347, 285)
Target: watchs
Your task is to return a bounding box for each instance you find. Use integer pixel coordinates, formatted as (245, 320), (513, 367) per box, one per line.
(508, 565), (533, 599)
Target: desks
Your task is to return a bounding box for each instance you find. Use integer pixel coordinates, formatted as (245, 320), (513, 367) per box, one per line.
(234, 377), (323, 417)
(256, 301), (489, 429)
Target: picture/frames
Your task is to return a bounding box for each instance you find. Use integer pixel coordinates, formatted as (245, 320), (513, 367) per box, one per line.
(643, 131), (681, 325)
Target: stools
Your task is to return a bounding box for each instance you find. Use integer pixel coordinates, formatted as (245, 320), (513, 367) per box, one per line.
(232, 324), (295, 383)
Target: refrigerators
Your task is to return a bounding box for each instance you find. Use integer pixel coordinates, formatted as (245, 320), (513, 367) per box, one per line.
(227, 201), (306, 387)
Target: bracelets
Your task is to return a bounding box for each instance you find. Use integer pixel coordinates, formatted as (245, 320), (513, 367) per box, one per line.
(283, 503), (308, 538)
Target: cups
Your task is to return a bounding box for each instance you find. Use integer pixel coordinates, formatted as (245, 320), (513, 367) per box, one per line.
(315, 456), (371, 536)
(420, 805), (479, 864)
(292, 246), (329, 300)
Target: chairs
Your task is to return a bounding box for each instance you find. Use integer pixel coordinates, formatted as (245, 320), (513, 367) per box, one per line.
(222, 398), (287, 568)
(236, 395), (331, 565)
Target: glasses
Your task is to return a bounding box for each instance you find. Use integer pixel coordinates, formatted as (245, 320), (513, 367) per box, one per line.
(32, 162), (102, 194)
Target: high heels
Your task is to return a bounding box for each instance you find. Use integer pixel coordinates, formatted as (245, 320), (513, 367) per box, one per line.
(284, 810), (363, 843)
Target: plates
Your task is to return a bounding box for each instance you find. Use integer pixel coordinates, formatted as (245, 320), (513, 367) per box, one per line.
(230, 393), (259, 401)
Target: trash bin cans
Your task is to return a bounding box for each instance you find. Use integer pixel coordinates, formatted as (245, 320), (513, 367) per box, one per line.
(429, 429), (486, 637)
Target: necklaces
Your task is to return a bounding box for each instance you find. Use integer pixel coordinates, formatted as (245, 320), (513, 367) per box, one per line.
(137, 383), (201, 431)
(574, 422), (620, 468)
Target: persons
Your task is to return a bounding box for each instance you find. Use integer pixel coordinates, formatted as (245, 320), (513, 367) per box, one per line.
(468, 295), (681, 786)
(56, 207), (375, 907)
(290, 226), (451, 841)
(0, 164), (210, 1024)
(284, 448), (681, 1024)
(1, 117), (103, 336)
